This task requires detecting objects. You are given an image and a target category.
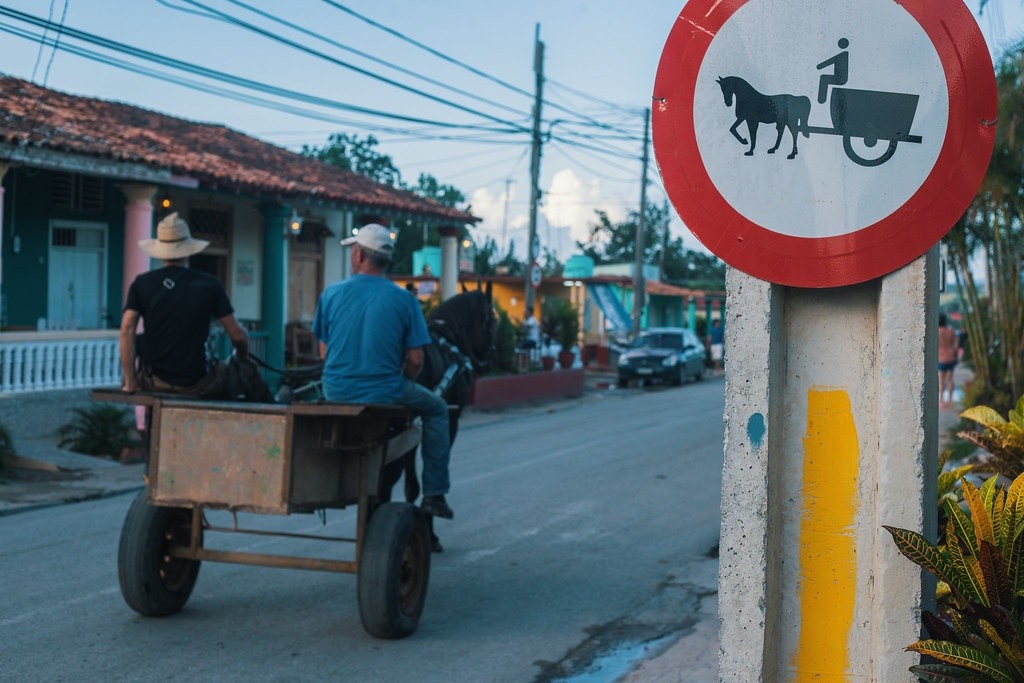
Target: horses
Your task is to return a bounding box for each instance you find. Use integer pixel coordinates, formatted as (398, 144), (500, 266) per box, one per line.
(367, 278), (499, 554)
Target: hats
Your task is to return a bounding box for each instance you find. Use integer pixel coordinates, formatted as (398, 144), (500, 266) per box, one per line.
(138, 211), (210, 260)
(340, 223), (396, 255)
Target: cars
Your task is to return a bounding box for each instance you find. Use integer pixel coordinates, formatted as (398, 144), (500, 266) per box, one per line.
(616, 326), (707, 389)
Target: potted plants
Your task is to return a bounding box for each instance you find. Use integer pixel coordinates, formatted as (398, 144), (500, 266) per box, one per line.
(541, 337), (555, 370)
(59, 404), (135, 463)
(540, 297), (580, 369)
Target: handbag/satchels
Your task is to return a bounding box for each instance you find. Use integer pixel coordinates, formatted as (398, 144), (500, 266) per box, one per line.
(218, 357), (272, 402)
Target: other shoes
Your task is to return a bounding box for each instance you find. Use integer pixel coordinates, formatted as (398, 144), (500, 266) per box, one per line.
(420, 494), (454, 520)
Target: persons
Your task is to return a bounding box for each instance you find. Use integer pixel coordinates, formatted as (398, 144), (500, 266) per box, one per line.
(705, 309), (723, 377)
(119, 211), (294, 405)
(938, 313), (959, 413)
(308, 222), (454, 520)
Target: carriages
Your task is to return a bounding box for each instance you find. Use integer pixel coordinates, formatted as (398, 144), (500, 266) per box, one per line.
(86, 273), (502, 640)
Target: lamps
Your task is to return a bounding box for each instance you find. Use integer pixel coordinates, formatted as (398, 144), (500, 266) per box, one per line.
(389, 219), (399, 246)
(286, 210), (304, 233)
(461, 231), (474, 253)
